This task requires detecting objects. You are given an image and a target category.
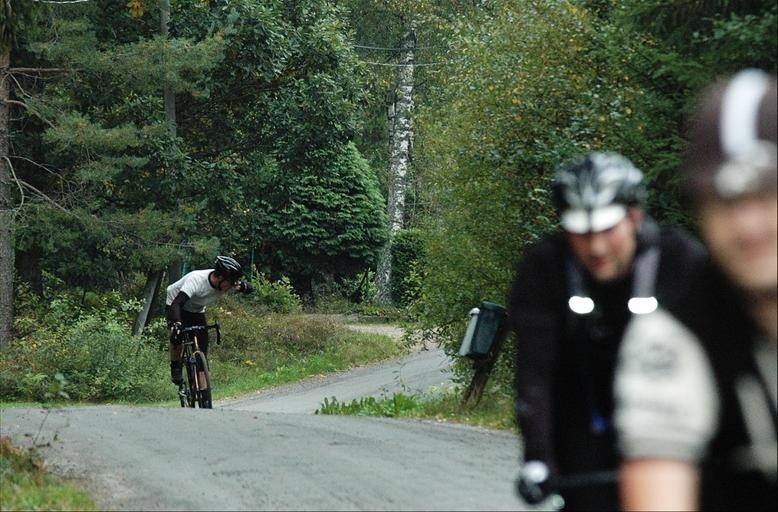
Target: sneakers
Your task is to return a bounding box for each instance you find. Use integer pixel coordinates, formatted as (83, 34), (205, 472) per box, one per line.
(170, 363), (184, 385)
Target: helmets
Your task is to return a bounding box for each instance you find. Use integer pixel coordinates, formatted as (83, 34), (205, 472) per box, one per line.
(551, 151), (644, 237)
(686, 67), (778, 199)
(214, 255), (243, 283)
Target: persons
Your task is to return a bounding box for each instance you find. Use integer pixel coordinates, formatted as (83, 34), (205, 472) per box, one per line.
(164, 254), (256, 409)
(610, 65), (777, 512)
(508, 147), (708, 512)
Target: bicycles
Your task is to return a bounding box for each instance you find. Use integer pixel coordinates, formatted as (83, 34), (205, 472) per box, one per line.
(173, 318), (221, 408)
(514, 463), (620, 511)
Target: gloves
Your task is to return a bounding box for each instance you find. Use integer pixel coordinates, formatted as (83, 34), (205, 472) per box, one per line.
(239, 281), (258, 295)
(169, 320), (183, 342)
